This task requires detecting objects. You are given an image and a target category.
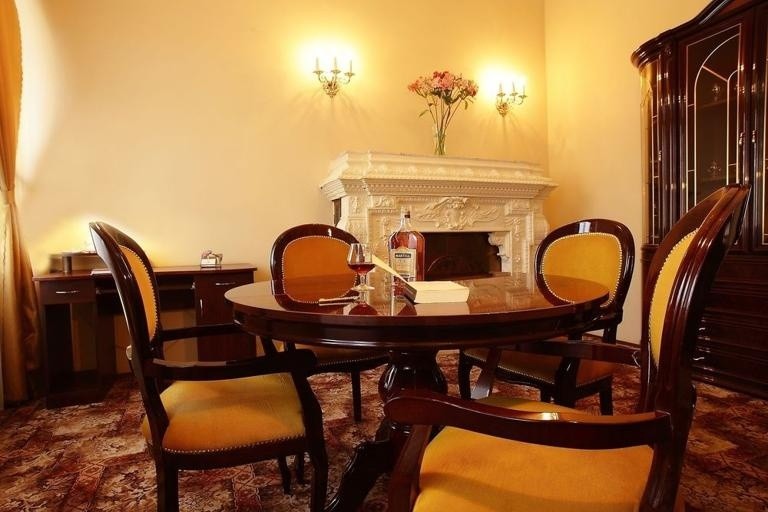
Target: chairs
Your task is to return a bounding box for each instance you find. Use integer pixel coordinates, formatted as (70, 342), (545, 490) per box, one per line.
(270, 224), (390, 422)
(89, 221), (328, 512)
(327, 184), (751, 512)
(459, 218), (635, 415)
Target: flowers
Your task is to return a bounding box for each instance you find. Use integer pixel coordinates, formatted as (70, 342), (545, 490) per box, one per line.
(407, 69), (479, 154)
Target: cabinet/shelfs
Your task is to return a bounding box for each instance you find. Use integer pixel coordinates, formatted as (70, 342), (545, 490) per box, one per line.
(32, 262), (257, 410)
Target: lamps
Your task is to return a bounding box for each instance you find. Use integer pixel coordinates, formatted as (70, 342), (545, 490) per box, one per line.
(496, 80), (527, 115)
(313, 56), (355, 97)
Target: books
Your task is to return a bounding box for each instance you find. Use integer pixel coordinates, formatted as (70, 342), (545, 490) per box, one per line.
(51, 253), (108, 271)
(372, 255), (470, 304)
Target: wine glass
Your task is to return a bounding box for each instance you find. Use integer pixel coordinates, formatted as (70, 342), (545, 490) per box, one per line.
(347, 243), (376, 292)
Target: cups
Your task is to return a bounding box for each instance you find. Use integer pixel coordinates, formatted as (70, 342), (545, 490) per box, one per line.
(62, 256), (72, 274)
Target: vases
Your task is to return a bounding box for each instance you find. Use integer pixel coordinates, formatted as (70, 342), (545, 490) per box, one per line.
(433, 126), (447, 156)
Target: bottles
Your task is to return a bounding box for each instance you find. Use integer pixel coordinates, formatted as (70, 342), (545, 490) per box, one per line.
(388, 210), (425, 298)
